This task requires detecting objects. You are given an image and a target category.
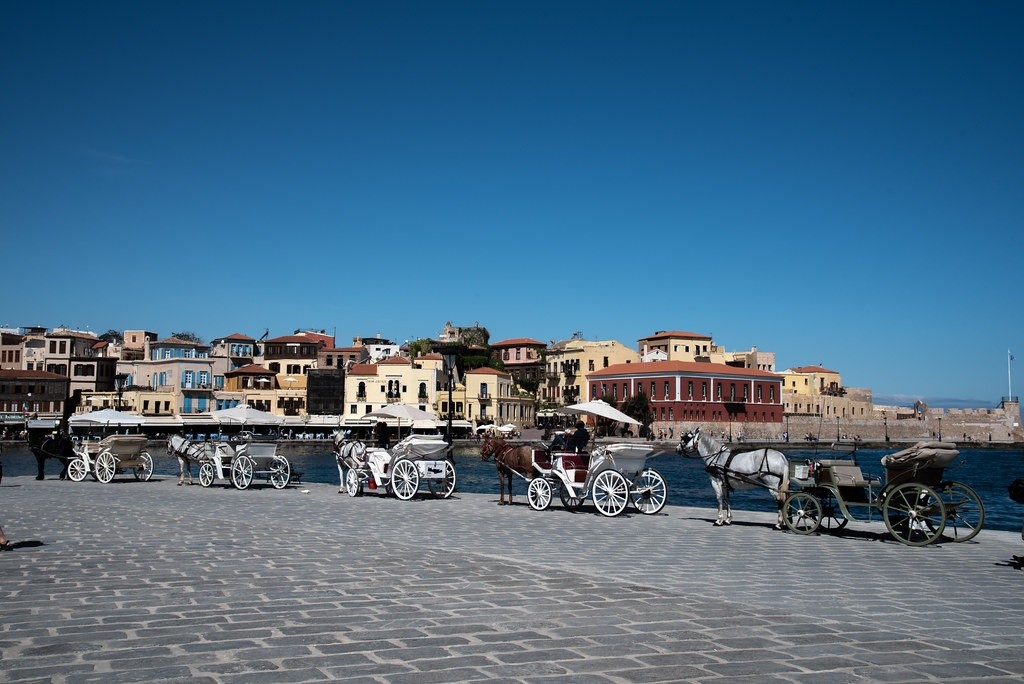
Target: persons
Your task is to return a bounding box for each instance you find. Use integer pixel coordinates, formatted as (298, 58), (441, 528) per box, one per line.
(738, 431), (744, 442)
(660, 428), (673, 439)
(805, 432), (816, 441)
(963, 433), (966, 441)
(853, 435), (861, 442)
(932, 431), (936, 440)
(842, 434), (848, 439)
(782, 431), (787, 442)
(721, 431), (725, 439)
(552, 421), (591, 451)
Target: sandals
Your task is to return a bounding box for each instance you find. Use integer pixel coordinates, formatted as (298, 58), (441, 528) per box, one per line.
(0, 540), (12, 552)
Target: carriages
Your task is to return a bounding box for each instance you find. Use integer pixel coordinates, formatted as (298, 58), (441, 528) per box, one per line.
(165, 404), (291, 489)
(24, 424), (154, 484)
(330, 403), (458, 503)
(478, 400), (670, 518)
(675, 426), (986, 548)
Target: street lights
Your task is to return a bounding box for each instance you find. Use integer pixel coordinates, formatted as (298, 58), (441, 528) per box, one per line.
(440, 348), (457, 492)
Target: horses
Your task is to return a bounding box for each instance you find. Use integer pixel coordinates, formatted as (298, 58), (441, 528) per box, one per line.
(332, 429), (368, 493)
(480, 437), (540, 505)
(675, 427), (789, 530)
(19, 430), (77, 480)
(167, 435), (212, 486)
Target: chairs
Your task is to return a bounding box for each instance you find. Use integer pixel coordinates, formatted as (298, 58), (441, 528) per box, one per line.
(818, 442), (856, 467)
(550, 435), (589, 466)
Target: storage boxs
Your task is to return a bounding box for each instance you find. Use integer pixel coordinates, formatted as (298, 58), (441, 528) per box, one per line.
(795, 464), (809, 479)
(415, 461), (445, 479)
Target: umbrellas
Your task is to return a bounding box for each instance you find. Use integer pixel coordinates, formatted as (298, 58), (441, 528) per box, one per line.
(359, 402), (437, 438)
(213, 403), (285, 443)
(69, 408), (144, 425)
(554, 399), (644, 452)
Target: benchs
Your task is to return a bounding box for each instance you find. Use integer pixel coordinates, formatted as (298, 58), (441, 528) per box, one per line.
(587, 444), (654, 474)
(217, 454), (233, 467)
(880, 441), (960, 486)
(97, 434), (148, 461)
(374, 452), (391, 474)
(236, 443), (277, 467)
(87, 446), (98, 460)
(557, 456), (589, 482)
(387, 434), (449, 470)
(830, 465), (883, 488)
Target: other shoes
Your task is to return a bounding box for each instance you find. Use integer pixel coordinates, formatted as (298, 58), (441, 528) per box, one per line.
(543, 449), (550, 456)
